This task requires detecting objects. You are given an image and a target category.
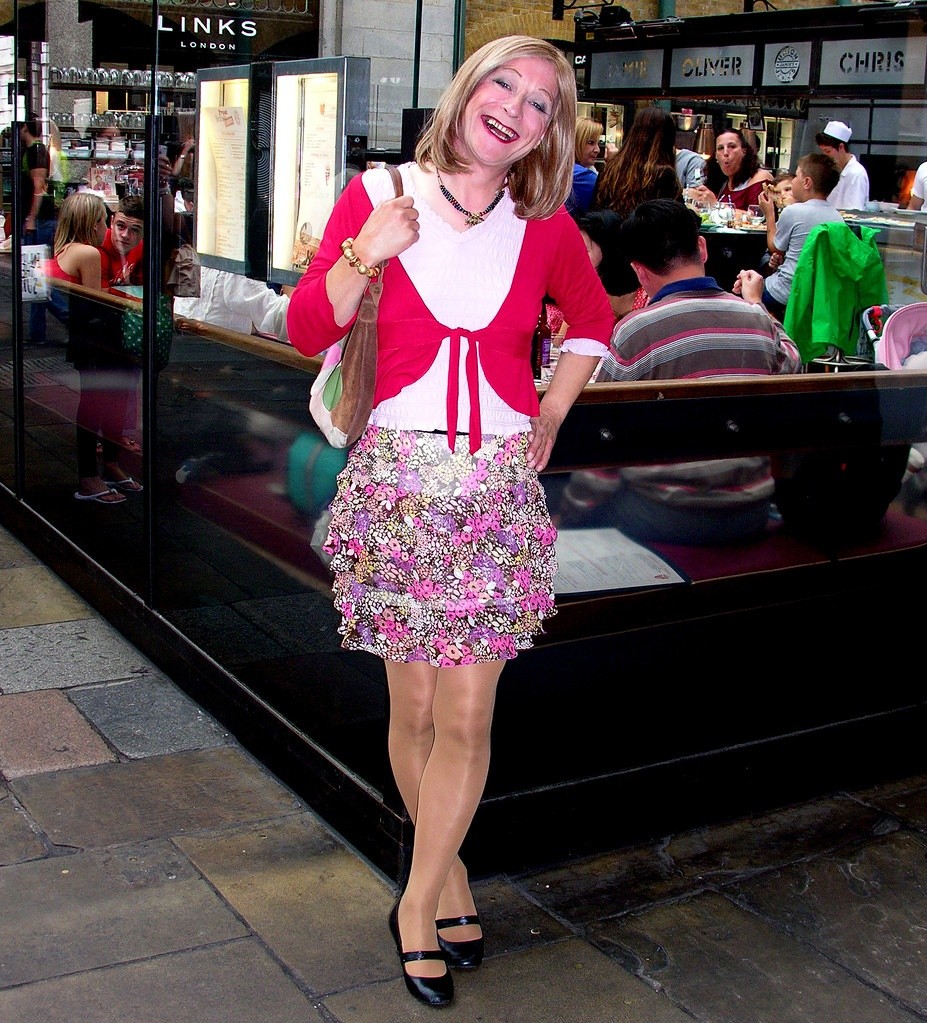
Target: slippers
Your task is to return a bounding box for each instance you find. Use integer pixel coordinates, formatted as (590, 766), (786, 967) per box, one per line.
(74, 487), (127, 504)
(102, 475), (144, 492)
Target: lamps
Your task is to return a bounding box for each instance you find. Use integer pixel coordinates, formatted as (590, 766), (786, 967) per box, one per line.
(553, 0), (634, 27)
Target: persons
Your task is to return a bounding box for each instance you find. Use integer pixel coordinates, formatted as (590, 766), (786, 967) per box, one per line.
(169, 262), (303, 347)
(536, 107), (870, 374)
(0, 113), (198, 293)
(545, 200), (801, 548)
(286, 37), (614, 1007)
(905, 162), (927, 212)
(68, 290), (175, 506)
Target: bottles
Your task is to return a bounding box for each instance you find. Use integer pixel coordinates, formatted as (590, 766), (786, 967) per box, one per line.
(531, 297), (551, 381)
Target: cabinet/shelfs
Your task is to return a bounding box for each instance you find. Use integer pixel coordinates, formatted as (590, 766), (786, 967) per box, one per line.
(17, 79), (197, 220)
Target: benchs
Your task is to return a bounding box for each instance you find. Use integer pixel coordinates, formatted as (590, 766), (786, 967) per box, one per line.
(0, 257), (927, 644)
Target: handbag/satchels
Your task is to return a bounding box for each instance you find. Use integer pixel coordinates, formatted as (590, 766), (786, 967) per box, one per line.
(167, 236), (202, 297)
(309, 159), (404, 448)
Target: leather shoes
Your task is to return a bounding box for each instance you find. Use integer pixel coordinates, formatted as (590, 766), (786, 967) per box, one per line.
(387, 897), (456, 1003)
(436, 914), (484, 970)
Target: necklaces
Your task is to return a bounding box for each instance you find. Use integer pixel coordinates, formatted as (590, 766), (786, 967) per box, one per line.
(437, 167), (506, 227)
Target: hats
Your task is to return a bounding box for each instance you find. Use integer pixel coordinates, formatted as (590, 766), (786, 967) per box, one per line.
(823, 120), (853, 143)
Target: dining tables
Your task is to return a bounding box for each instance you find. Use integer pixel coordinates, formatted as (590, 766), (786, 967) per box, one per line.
(700, 229), (768, 271)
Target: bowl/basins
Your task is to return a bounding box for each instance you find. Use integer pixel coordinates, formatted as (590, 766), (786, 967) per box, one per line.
(671, 114), (702, 133)
(878, 202), (899, 213)
(865, 201), (884, 213)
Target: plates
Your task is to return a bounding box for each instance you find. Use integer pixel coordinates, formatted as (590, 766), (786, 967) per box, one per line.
(741, 227), (768, 232)
(894, 208), (927, 215)
(699, 223), (717, 231)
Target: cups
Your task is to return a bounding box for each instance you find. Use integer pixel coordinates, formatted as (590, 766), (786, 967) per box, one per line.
(683, 197), (736, 221)
(747, 204), (765, 225)
(550, 331), (563, 355)
(49, 111), (144, 128)
(50, 66), (196, 87)
(541, 364), (557, 385)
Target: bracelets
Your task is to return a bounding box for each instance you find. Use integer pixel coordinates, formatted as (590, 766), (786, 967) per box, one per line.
(341, 237), (381, 278)
